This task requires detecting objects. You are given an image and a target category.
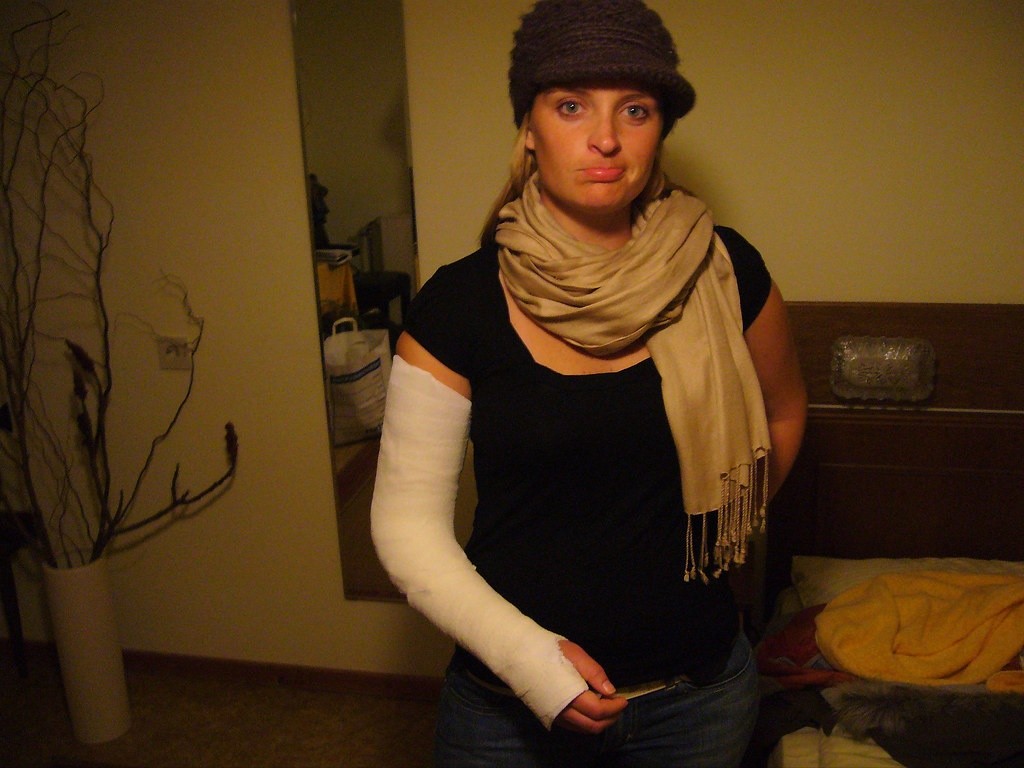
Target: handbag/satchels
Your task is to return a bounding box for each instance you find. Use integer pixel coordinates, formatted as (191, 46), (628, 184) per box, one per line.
(323, 316), (392, 448)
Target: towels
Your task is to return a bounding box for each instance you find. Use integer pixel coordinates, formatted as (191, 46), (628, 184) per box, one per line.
(812, 568), (1024, 693)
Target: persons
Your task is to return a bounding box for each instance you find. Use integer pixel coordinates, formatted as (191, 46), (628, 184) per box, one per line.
(369, 0), (808, 768)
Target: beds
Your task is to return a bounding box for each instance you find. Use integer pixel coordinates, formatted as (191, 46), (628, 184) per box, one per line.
(741, 408), (1024, 768)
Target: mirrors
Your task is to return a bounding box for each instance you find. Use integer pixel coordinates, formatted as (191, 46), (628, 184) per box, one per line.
(289, 0), (421, 605)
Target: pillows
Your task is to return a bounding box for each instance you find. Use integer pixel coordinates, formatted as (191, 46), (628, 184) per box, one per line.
(790, 555), (1024, 607)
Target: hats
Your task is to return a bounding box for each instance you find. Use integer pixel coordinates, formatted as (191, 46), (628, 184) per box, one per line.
(508, 0), (696, 130)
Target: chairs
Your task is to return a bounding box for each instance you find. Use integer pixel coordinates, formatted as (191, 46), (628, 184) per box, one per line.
(0, 401), (54, 679)
(312, 203), (411, 348)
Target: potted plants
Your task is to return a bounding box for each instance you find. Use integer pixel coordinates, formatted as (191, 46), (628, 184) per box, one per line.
(0, 0), (238, 747)
